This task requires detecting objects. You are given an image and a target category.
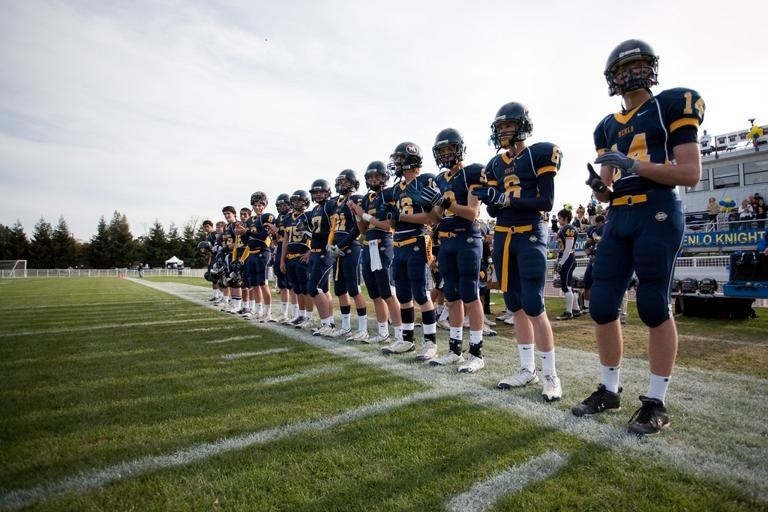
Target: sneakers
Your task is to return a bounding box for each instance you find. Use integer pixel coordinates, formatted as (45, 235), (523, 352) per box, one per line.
(555, 306), (589, 320)
(414, 341), (437, 360)
(542, 369), (562, 401)
(628, 396), (668, 434)
(429, 350), (465, 365)
(498, 367), (539, 389)
(435, 313), (515, 336)
(210, 295), (415, 354)
(572, 385), (622, 417)
(457, 354), (484, 373)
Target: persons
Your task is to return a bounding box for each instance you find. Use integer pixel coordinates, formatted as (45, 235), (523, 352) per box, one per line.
(572, 38), (705, 437)
(137, 264), (143, 277)
(404, 128), (487, 373)
(552, 195), (627, 322)
(471, 103), (563, 401)
(708, 193), (767, 231)
(746, 118), (763, 144)
(701, 130), (711, 157)
(479, 219), (515, 335)
(199, 141), (448, 361)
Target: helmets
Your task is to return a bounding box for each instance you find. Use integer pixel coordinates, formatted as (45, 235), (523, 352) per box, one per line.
(553, 275), (584, 289)
(604, 40), (659, 96)
(365, 161), (389, 191)
(290, 190), (309, 211)
(432, 128), (466, 169)
(491, 102), (533, 149)
(386, 142), (423, 177)
(197, 240), (211, 254)
(251, 192), (268, 207)
(309, 179), (331, 202)
(673, 278), (718, 293)
(210, 264), (246, 288)
(275, 193), (290, 214)
(335, 170), (359, 194)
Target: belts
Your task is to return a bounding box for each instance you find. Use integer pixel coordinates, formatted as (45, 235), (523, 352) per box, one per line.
(287, 254), (301, 260)
(439, 232), (455, 237)
(335, 246), (349, 282)
(310, 249), (321, 252)
(393, 237), (417, 246)
(612, 194), (647, 205)
(495, 224), (532, 293)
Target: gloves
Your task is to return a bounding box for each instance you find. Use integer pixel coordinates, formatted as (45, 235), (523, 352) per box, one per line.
(594, 149), (634, 173)
(420, 186), (444, 206)
(377, 204), (400, 222)
(586, 163), (607, 194)
(405, 184), (429, 209)
(471, 181), (507, 209)
